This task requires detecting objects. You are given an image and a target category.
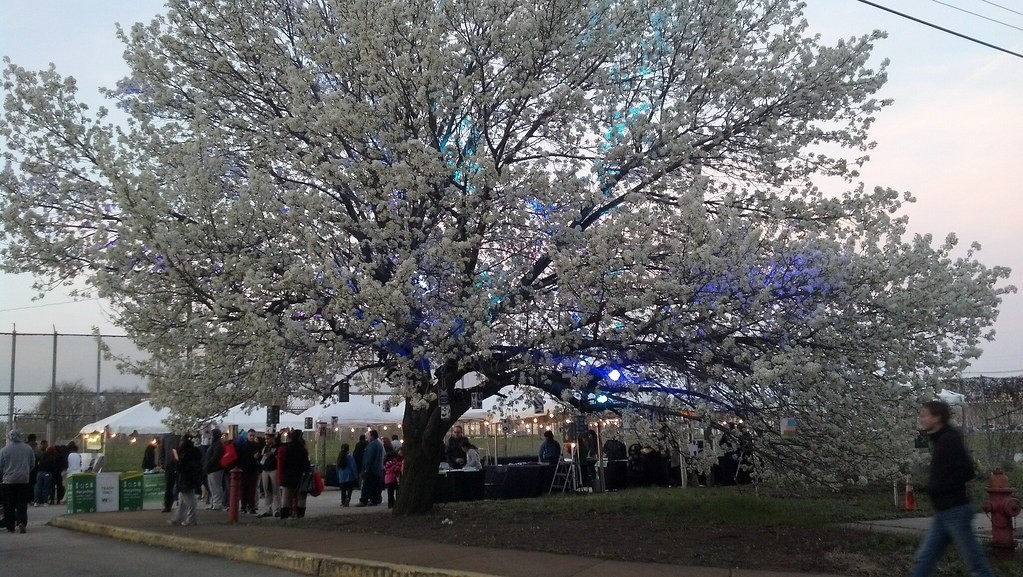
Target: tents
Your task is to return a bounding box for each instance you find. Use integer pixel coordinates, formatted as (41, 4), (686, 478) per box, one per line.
(207, 400), (306, 432)
(576, 369), (1023, 487)
(394, 399), (493, 466)
(494, 391), (576, 465)
(81, 399), (214, 453)
(301, 395), (401, 425)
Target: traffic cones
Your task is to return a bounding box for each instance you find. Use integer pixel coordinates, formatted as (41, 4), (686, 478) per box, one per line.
(903, 472), (920, 511)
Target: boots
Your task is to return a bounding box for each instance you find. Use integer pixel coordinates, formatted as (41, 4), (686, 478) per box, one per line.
(281, 508), (289, 519)
(297, 506), (306, 519)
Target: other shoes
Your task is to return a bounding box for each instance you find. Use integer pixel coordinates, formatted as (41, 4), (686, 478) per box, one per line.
(3, 528), (16, 533)
(206, 506), (222, 509)
(249, 510), (258, 514)
(43, 503), (49, 506)
(34, 502), (41, 507)
(162, 507), (171, 512)
(239, 510), (247, 513)
(262, 511), (273, 517)
(57, 499), (60, 504)
(168, 519), (181, 525)
(340, 496), (397, 509)
(274, 511), (280, 517)
(180, 520), (197, 526)
(19, 523), (26, 534)
(50, 499), (54, 505)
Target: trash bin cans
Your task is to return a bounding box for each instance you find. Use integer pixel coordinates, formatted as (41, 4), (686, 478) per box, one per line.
(96, 472), (122, 512)
(120, 473), (144, 511)
(66, 472), (97, 513)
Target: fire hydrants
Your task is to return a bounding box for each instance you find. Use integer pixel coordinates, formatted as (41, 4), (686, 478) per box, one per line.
(981, 466), (1021, 561)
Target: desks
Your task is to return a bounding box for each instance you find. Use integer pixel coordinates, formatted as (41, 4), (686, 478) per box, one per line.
(325, 465), (361, 490)
(436, 453), (758, 504)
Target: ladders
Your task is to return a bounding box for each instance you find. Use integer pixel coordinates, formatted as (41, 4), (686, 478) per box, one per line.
(549, 442), (576, 495)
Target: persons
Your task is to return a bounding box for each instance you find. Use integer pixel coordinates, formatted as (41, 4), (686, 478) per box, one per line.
(910, 400), (991, 577)
(538, 423), (754, 492)
(159, 428), (314, 526)
(336, 430), (404, 508)
(441, 426), (482, 469)
(0, 430), (79, 533)
(141, 437), (160, 472)
